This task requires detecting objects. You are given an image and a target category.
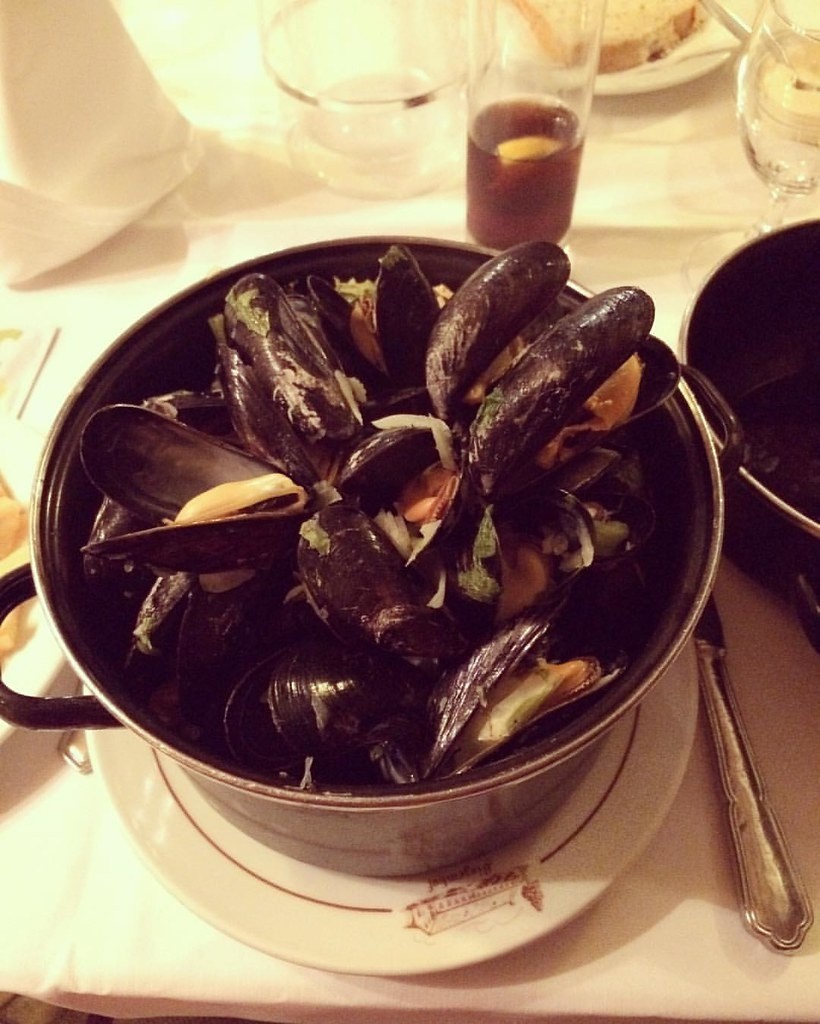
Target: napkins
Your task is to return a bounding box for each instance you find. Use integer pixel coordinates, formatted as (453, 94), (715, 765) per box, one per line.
(0, 0), (200, 286)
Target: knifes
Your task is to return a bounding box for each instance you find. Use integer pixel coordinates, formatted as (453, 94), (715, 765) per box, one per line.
(692, 591), (813, 950)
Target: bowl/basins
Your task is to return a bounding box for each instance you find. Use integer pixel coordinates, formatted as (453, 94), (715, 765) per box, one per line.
(678, 216), (820, 633)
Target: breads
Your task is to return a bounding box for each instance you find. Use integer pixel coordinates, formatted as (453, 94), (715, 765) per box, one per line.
(512, 0), (703, 77)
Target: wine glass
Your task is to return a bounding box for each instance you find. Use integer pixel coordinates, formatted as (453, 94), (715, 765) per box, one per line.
(685, 0), (820, 293)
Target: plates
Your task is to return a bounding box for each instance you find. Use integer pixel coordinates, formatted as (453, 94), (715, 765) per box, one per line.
(495, 0), (761, 96)
(80, 642), (699, 975)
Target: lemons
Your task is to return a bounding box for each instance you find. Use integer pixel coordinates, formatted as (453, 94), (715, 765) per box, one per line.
(497, 135), (565, 167)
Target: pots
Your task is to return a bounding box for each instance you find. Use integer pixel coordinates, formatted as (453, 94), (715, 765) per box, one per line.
(0, 234), (744, 882)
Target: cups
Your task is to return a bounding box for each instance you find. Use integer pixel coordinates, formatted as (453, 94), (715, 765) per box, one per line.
(256, 0), (470, 201)
(465, 0), (607, 261)
